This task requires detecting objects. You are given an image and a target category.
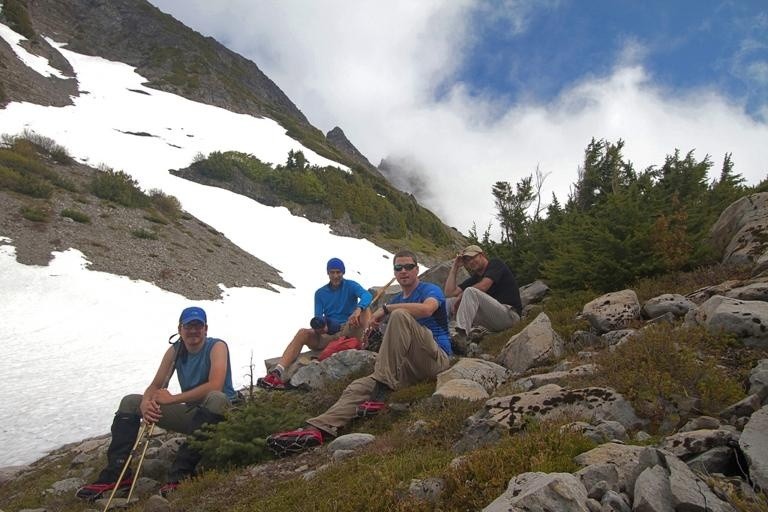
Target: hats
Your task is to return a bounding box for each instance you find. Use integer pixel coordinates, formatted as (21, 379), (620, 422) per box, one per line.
(460, 245), (483, 259)
(179, 307), (206, 325)
(327, 258), (345, 274)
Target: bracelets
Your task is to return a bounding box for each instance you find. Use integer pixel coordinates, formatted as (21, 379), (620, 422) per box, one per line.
(382, 302), (389, 314)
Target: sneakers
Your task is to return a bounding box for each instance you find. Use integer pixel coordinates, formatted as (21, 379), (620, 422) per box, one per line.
(265, 422), (323, 459)
(362, 326), (383, 353)
(75, 476), (132, 499)
(357, 382), (394, 416)
(448, 333), (467, 352)
(257, 370), (286, 390)
(159, 483), (180, 497)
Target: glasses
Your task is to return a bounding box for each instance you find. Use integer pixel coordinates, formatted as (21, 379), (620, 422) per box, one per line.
(180, 322), (204, 329)
(394, 264), (417, 271)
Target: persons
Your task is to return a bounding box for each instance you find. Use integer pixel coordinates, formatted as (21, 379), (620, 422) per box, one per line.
(264, 250), (454, 458)
(444, 245), (522, 354)
(68, 307), (234, 501)
(257, 257), (372, 389)
